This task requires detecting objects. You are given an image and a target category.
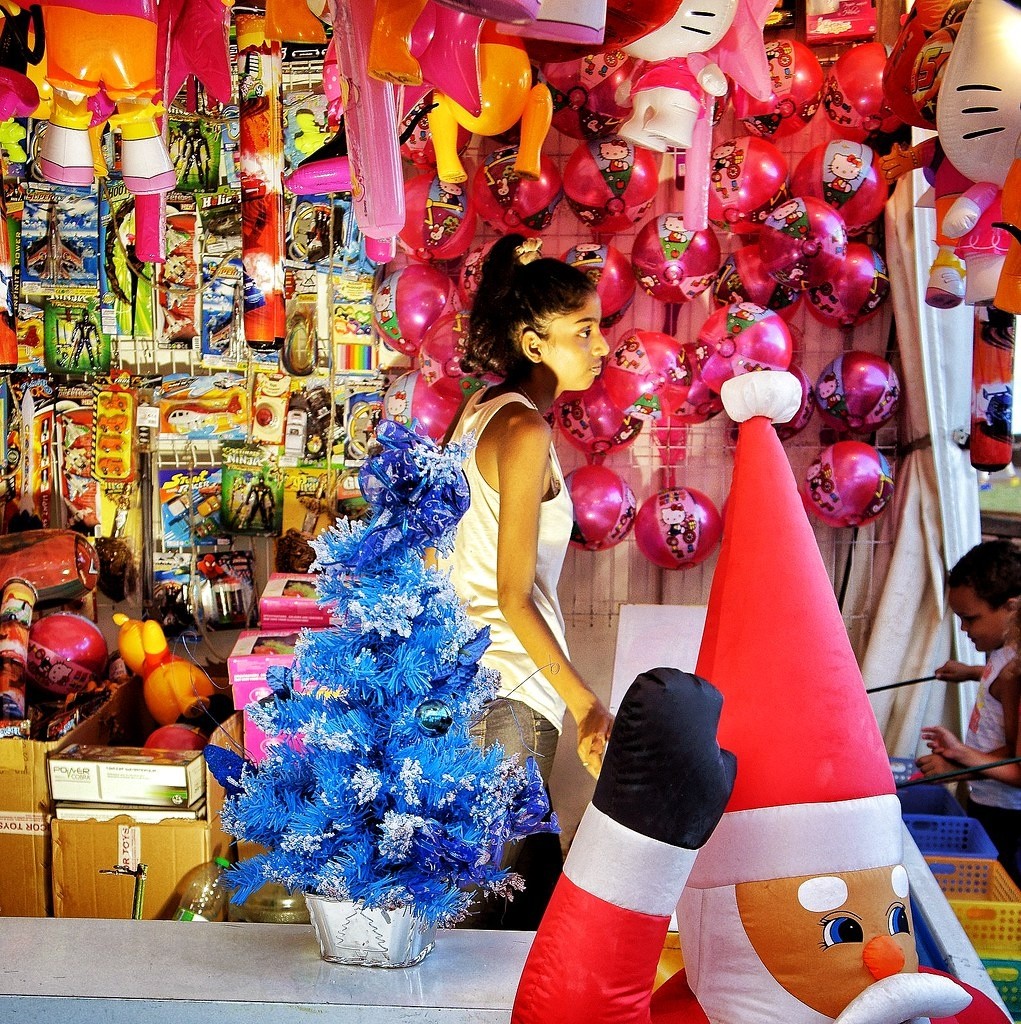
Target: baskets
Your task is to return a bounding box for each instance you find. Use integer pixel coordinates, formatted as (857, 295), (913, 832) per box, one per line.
(888, 756), (1021, 1024)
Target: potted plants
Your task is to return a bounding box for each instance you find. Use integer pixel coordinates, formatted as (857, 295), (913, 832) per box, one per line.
(217, 417), (561, 970)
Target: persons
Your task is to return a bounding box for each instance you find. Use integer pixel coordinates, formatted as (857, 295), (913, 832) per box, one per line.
(914, 540), (1021, 888)
(425, 235), (615, 931)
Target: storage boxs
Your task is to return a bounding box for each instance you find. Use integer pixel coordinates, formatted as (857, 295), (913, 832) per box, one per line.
(41, 711), (243, 918)
(54, 794), (208, 824)
(46, 743), (206, 807)
(232, 682), (350, 768)
(226, 626), (340, 685)
(258, 571), (360, 631)
(0, 675), (158, 918)
